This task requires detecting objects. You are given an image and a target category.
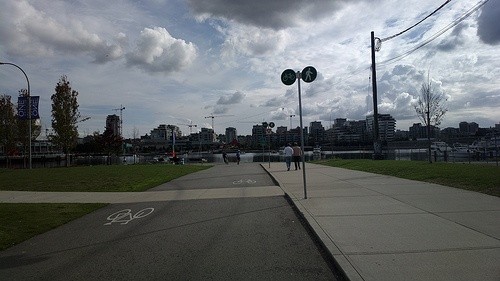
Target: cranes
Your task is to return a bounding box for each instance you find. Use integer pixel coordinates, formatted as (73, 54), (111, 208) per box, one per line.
(204, 112), (236, 132)
(176, 120), (197, 135)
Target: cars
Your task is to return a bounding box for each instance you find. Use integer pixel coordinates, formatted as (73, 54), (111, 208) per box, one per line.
(153, 157), (169, 163)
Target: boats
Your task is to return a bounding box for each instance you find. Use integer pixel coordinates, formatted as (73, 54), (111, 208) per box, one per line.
(451, 143), (472, 154)
(430, 142), (452, 153)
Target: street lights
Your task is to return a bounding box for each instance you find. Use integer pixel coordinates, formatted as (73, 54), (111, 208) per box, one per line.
(120, 107), (126, 138)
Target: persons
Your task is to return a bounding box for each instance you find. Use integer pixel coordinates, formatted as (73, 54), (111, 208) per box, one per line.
(283, 143), (294, 170)
(236, 150), (241, 165)
(292, 142), (302, 170)
(56, 154), (61, 167)
(70, 154), (73, 167)
(40, 155), (46, 168)
(222, 151), (229, 163)
